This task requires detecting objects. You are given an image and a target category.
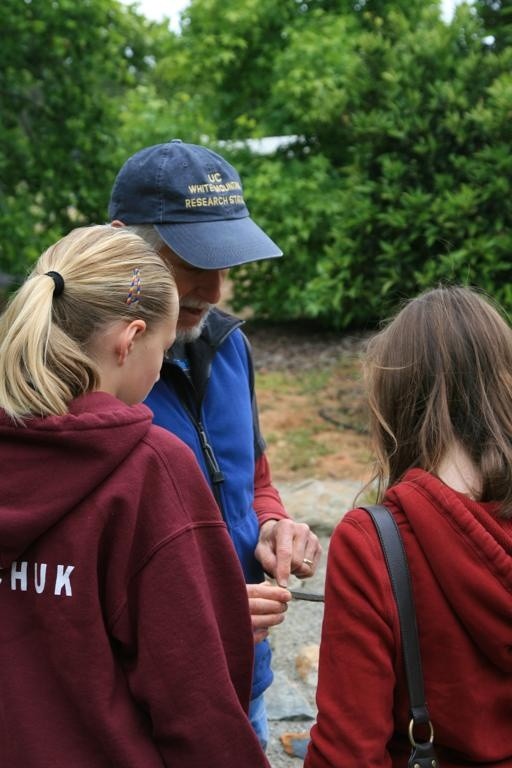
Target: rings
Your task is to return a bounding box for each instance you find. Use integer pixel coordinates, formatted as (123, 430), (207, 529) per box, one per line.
(303, 558), (313, 567)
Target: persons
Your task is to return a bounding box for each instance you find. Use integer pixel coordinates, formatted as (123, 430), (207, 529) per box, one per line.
(303, 285), (512, 768)
(108, 138), (322, 753)
(0, 222), (272, 768)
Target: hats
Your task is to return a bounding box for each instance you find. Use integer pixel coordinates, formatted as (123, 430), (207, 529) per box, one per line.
(107, 138), (284, 272)
